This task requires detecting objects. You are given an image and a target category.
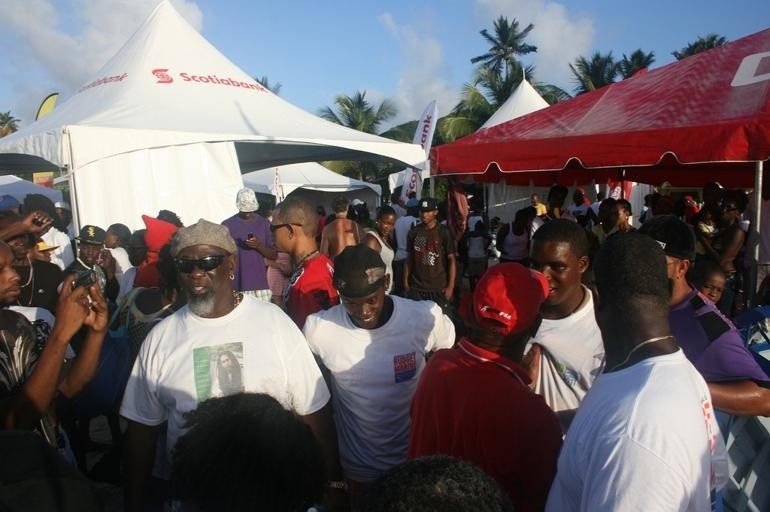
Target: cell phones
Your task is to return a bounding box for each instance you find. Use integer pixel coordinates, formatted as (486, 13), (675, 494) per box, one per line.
(248, 233), (253, 240)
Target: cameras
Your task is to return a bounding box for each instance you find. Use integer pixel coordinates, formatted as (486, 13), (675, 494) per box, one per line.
(56, 270), (97, 311)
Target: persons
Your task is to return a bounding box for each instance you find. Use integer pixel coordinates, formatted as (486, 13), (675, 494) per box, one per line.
(1, 187), (768, 512)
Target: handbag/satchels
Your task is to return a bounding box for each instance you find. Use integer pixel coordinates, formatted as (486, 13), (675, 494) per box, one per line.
(69, 287), (148, 420)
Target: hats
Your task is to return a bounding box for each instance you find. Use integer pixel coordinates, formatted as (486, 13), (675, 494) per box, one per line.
(54, 201), (71, 211)
(168, 218), (238, 258)
(142, 215), (177, 252)
(236, 188), (259, 213)
(332, 243), (386, 298)
(473, 262), (550, 335)
(36, 242), (60, 252)
(637, 215), (695, 262)
(74, 226), (106, 246)
(418, 197), (438, 212)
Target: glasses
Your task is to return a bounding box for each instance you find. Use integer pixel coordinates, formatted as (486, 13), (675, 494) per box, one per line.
(270, 223), (302, 232)
(171, 253), (231, 273)
